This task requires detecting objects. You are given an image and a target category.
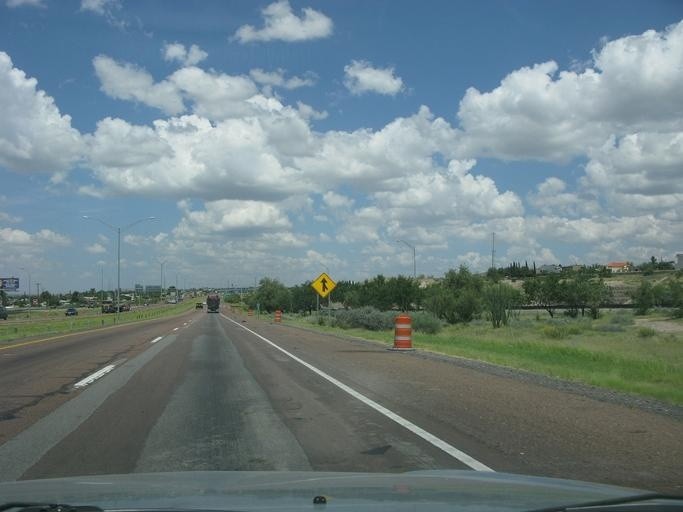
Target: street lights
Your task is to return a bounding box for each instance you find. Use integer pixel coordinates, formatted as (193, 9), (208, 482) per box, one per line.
(396, 239), (415, 279)
(175, 272), (180, 303)
(19, 266), (31, 322)
(155, 259), (167, 303)
(81, 215), (155, 313)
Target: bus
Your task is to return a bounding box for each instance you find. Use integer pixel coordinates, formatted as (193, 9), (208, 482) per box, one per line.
(207, 294), (220, 313)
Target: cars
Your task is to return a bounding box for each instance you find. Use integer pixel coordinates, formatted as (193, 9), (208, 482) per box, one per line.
(196, 301), (206, 310)
(0, 305), (8, 320)
(65, 308), (78, 316)
(102, 300), (130, 313)
